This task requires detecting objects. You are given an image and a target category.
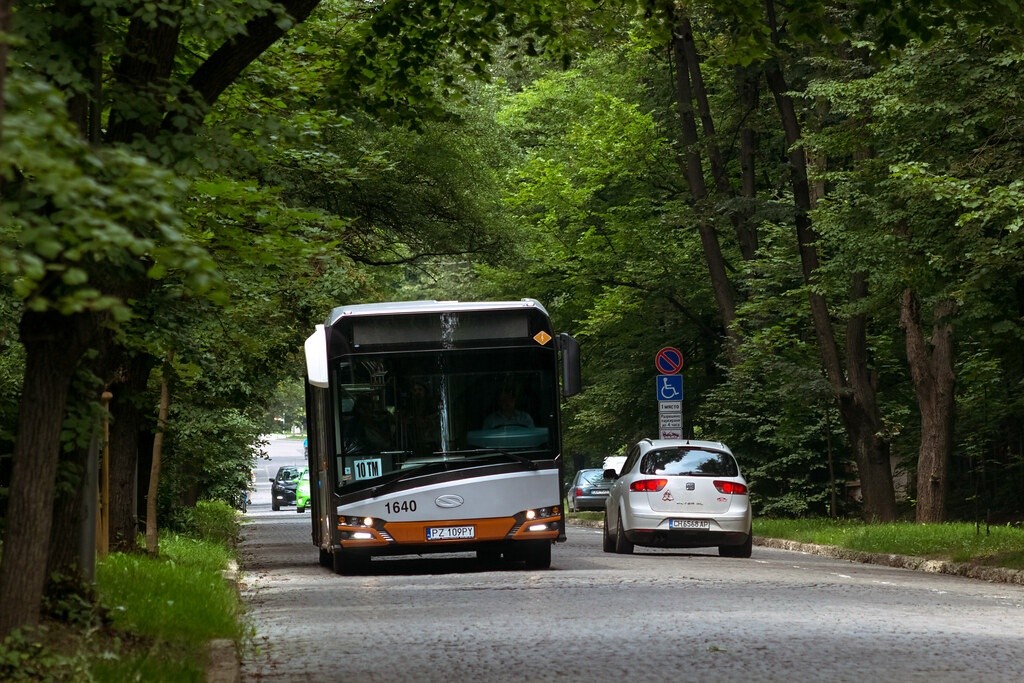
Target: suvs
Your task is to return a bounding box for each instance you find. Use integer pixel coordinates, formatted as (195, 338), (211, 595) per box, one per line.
(268, 465), (306, 511)
(601, 438), (755, 556)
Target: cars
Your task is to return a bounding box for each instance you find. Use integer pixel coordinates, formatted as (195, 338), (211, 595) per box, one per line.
(293, 469), (311, 513)
(564, 469), (620, 512)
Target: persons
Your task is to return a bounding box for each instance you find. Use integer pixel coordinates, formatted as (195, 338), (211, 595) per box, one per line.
(246, 469), (256, 504)
(483, 391), (535, 431)
(406, 381), (438, 445)
(303, 438), (308, 459)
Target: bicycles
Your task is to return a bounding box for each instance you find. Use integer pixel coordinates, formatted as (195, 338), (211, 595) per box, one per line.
(238, 487), (255, 512)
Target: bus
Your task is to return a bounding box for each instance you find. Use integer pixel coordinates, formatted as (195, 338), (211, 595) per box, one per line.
(303, 297), (582, 576)
